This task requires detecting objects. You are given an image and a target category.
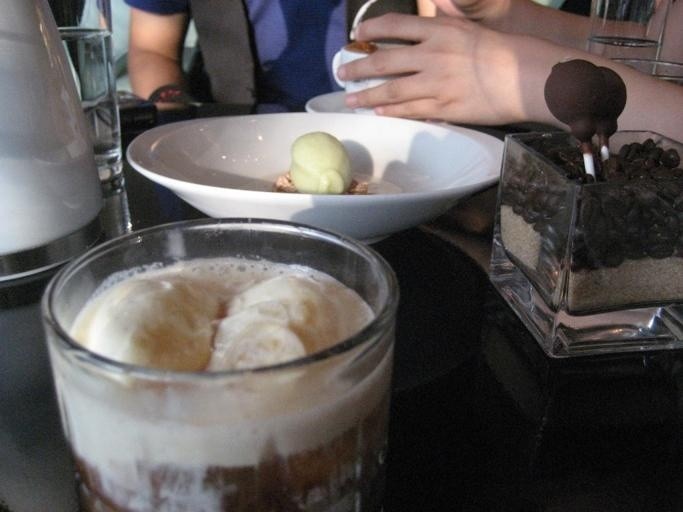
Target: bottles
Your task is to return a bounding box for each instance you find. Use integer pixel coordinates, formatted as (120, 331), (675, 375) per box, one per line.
(1, 1), (109, 285)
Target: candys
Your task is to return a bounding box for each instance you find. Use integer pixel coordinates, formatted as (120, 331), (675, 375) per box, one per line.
(545, 59), (627, 185)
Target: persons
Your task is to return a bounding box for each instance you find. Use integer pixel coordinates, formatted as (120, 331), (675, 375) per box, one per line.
(121, 1), (347, 112)
(333, 1), (682, 170)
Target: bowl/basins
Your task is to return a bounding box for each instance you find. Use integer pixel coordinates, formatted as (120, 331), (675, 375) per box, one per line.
(129, 113), (506, 246)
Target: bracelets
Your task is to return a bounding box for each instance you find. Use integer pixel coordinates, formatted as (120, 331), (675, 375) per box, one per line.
(146, 83), (189, 103)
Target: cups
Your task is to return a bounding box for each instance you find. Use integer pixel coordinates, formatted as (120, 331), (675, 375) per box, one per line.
(39, 216), (400, 511)
(59, 25), (121, 181)
(338, 40), (411, 114)
(585, 0), (683, 92)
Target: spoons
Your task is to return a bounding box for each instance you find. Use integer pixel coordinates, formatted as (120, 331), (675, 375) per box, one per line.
(349, 0), (378, 40)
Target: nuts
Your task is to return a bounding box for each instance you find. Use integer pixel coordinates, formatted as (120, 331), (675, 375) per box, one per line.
(500, 137), (683, 272)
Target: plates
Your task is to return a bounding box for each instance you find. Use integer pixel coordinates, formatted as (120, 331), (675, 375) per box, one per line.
(304, 88), (376, 118)
